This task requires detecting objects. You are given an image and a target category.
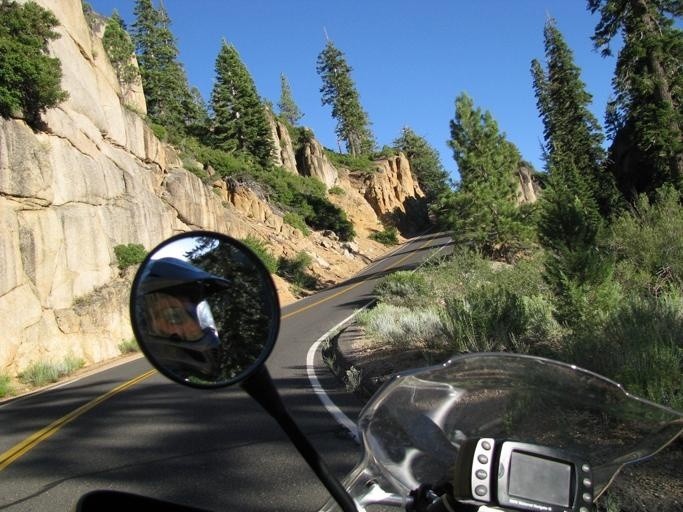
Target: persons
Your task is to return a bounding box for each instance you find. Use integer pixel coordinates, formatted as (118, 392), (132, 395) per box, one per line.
(204, 164), (214, 177)
(221, 176), (238, 205)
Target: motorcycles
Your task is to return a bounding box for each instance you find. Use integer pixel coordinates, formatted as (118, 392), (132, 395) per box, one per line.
(63, 227), (681, 512)
